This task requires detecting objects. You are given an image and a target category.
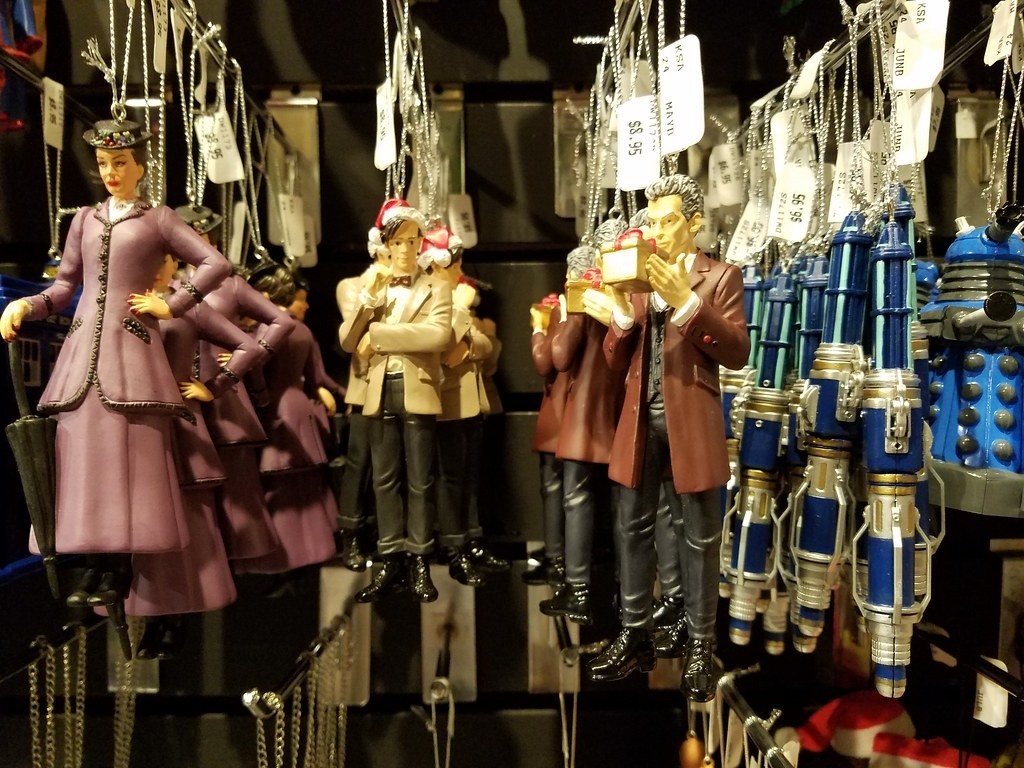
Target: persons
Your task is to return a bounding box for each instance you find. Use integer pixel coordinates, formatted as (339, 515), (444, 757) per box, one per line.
(0, 119), (752, 703)
